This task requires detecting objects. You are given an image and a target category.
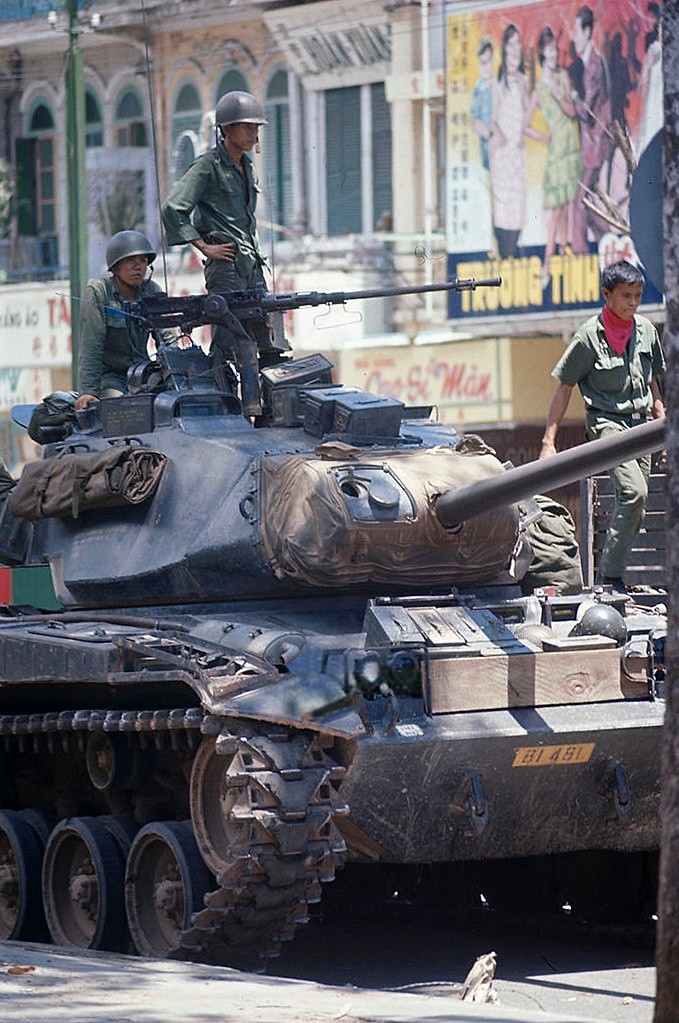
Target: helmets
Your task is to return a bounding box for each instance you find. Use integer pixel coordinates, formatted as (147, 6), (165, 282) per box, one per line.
(106, 230), (156, 271)
(568, 603), (627, 647)
(215, 90), (269, 127)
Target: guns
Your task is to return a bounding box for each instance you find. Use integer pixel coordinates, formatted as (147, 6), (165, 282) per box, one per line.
(112, 273), (503, 336)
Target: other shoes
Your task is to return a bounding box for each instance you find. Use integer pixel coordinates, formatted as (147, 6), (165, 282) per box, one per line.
(596, 571), (629, 595)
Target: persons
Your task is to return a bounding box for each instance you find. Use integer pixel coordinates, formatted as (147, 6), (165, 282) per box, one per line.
(75, 230), (183, 411)
(161, 91), (287, 364)
(538, 257), (667, 594)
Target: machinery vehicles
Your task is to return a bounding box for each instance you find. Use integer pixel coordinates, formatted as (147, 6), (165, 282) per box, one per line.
(0, 278), (668, 974)
